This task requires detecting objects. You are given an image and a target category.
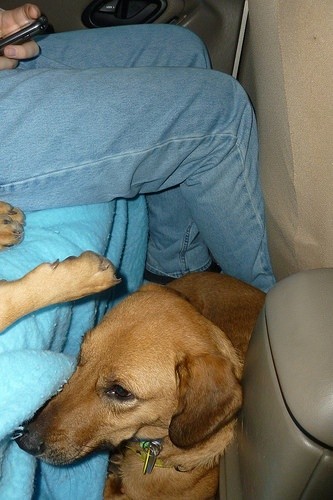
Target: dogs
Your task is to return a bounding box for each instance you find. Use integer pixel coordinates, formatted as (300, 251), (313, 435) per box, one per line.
(15, 272), (269, 500)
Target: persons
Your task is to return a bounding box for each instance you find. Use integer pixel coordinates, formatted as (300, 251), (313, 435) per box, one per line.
(1, 3), (274, 293)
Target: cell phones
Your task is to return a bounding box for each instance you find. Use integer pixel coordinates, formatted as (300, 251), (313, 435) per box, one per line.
(0, 15), (49, 51)
